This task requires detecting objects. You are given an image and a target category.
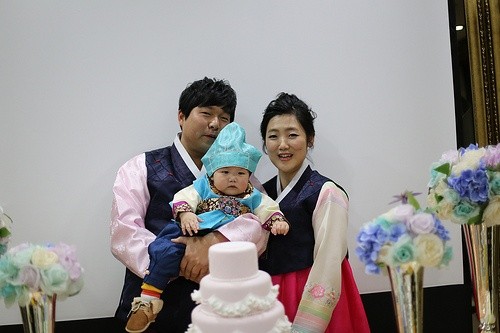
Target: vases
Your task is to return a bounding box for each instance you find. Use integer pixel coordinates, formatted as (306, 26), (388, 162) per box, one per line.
(19, 294), (57, 333)
(388, 266), (426, 333)
(460, 221), (500, 333)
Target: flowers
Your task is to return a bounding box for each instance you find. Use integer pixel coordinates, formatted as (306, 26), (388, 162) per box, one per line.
(0, 240), (85, 309)
(426, 142), (500, 227)
(353, 190), (454, 276)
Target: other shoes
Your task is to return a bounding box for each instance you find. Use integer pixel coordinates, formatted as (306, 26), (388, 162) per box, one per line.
(125, 297), (164, 333)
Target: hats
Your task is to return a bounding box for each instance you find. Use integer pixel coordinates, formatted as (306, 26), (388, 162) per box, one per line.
(201, 122), (262, 177)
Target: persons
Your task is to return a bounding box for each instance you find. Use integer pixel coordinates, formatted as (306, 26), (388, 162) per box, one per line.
(110, 76), (271, 333)
(259, 93), (372, 333)
(125, 122), (289, 333)
